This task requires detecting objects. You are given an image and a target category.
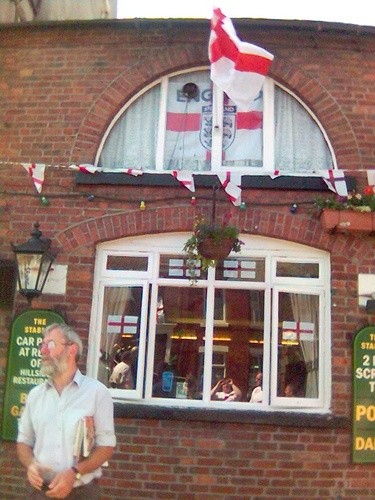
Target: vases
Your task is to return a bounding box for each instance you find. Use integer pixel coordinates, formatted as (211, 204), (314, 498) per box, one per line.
(198, 236), (236, 260)
(318, 208), (375, 235)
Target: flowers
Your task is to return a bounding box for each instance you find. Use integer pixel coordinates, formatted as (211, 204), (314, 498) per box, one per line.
(183, 213), (247, 288)
(312, 184), (375, 217)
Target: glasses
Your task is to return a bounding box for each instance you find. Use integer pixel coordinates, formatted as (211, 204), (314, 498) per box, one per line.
(37, 339), (74, 351)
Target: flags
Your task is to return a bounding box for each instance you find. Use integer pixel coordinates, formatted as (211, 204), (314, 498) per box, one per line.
(209, 7), (273, 109)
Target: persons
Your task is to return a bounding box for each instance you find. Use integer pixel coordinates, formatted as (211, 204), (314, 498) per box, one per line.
(185, 370), (201, 399)
(152, 362), (178, 398)
(285, 383), (294, 397)
(16, 323), (117, 500)
(250, 372), (263, 403)
(105, 344), (139, 389)
(208, 378), (241, 401)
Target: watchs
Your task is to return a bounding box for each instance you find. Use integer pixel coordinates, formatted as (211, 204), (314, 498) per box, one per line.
(71, 466), (82, 480)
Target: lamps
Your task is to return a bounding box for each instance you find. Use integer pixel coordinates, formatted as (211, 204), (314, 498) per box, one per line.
(8, 218), (60, 309)
